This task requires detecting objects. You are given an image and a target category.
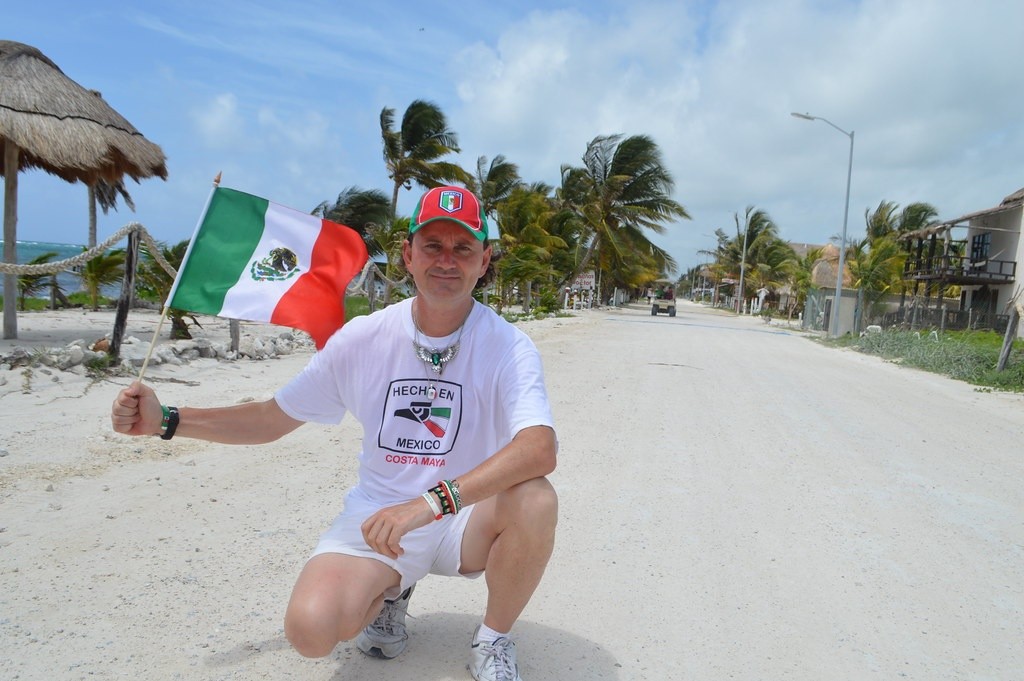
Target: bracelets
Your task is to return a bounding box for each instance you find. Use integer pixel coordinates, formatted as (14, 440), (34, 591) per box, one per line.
(161, 405), (180, 440)
(422, 479), (462, 520)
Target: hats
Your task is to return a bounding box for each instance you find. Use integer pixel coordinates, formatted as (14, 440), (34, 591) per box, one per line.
(408, 186), (488, 245)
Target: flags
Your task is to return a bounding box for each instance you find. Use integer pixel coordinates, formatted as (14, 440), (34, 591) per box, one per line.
(165, 182), (370, 351)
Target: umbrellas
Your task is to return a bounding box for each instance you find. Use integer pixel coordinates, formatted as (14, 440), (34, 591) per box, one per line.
(0, 39), (169, 338)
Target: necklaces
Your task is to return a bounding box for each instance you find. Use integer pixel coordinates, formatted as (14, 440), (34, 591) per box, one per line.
(412, 298), (470, 400)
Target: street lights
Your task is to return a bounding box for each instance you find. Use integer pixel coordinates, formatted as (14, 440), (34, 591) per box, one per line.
(789, 111), (855, 337)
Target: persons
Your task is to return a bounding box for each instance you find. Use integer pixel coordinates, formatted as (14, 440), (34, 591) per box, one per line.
(655, 286), (673, 300)
(647, 289), (652, 305)
(634, 286), (640, 303)
(114, 186), (558, 681)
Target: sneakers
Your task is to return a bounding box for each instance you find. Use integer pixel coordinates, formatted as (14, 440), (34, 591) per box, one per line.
(465, 623), (520, 681)
(355, 582), (416, 658)
